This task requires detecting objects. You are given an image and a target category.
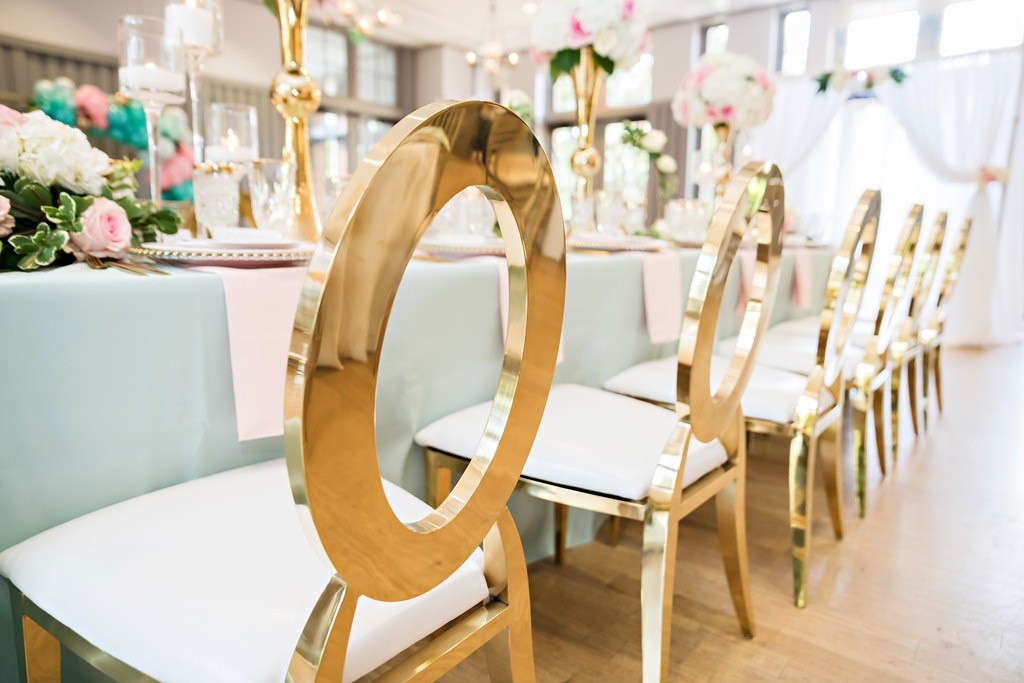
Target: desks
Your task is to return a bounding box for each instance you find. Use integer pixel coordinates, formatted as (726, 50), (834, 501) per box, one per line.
(0, 225), (844, 683)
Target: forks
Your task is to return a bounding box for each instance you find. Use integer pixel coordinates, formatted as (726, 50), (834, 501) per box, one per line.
(85, 255), (146, 276)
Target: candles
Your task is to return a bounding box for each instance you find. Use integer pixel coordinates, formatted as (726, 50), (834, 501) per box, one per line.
(162, 0), (224, 58)
(211, 103), (259, 164)
(117, 14), (186, 105)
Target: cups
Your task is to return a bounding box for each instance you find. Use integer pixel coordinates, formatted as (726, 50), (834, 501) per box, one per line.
(190, 101), (295, 232)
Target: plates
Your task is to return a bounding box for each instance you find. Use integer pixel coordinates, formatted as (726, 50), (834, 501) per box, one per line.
(568, 231), (671, 251)
(418, 244), (506, 263)
(125, 228), (316, 269)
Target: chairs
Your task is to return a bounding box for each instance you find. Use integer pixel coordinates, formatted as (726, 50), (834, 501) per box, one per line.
(805, 216), (973, 433)
(716, 203), (923, 520)
(773, 210), (950, 465)
(413, 156), (789, 683)
(602, 190), (882, 608)
(0, 99), (566, 683)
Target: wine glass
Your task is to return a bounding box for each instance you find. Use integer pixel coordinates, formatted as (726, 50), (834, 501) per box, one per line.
(117, 1), (223, 209)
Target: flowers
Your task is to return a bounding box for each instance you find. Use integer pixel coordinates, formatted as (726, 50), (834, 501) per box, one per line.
(811, 64), (907, 99)
(669, 49), (776, 130)
(621, 120), (679, 196)
(527, 0), (647, 85)
(500, 89), (533, 121)
(0, 105), (181, 271)
